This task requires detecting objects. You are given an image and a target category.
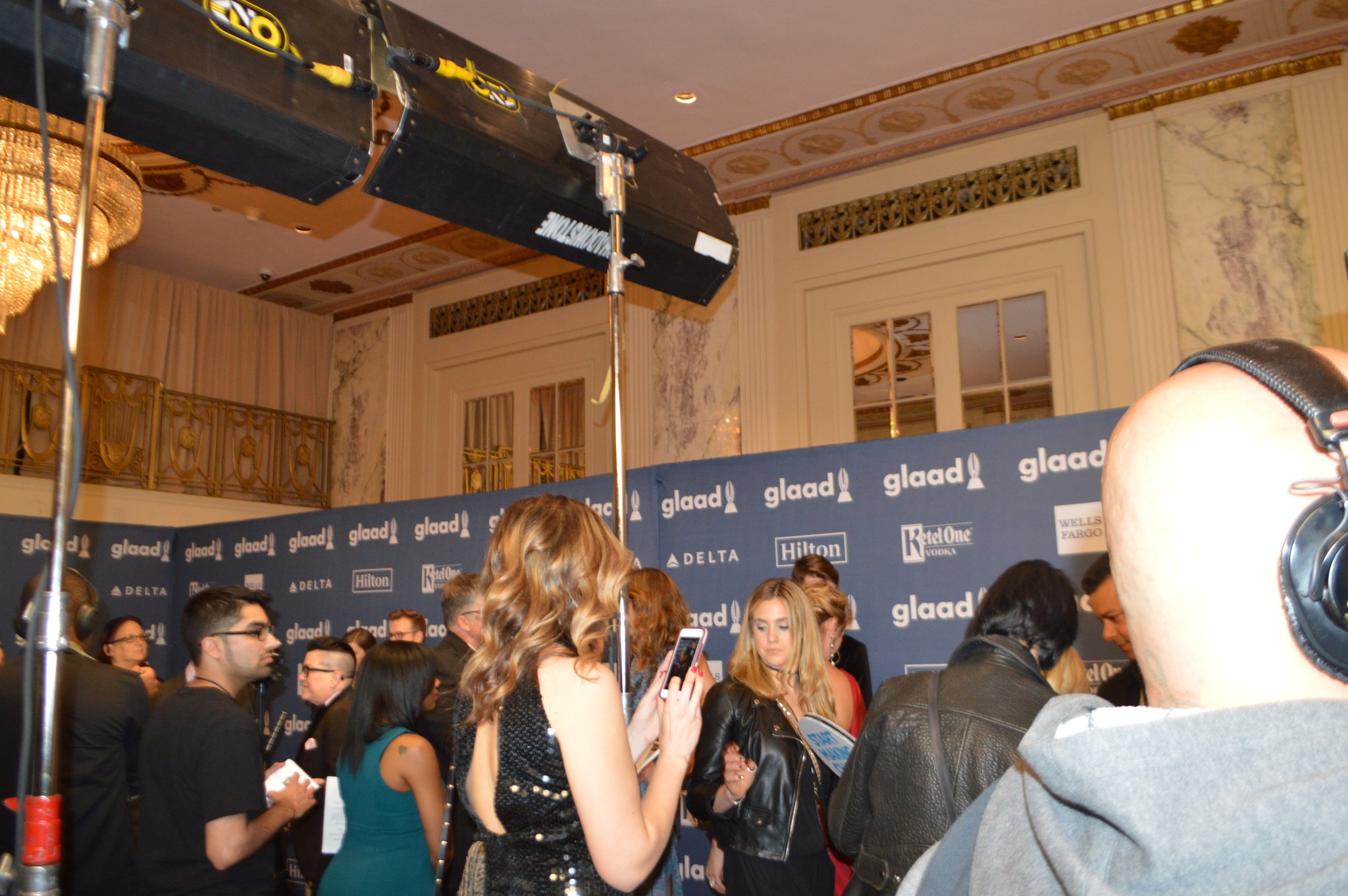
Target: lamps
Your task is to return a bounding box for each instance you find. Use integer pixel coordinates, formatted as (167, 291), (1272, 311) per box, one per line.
(0, 95), (144, 336)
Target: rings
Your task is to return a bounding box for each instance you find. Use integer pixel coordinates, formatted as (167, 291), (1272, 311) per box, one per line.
(747, 764), (757, 772)
(139, 671), (144, 674)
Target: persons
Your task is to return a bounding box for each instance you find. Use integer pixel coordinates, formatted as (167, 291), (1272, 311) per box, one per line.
(155, 657), (255, 717)
(1079, 546), (1143, 708)
(95, 616), (149, 671)
(417, 573), (484, 788)
(1041, 645), (1089, 697)
(624, 568), (716, 896)
(0, 566), (150, 896)
(827, 559), (1078, 896)
(388, 609), (426, 645)
(139, 584), (325, 896)
(895, 343), (1348, 896)
(343, 628), (376, 688)
(288, 638), (356, 896)
(800, 580), (868, 896)
(792, 554), (873, 711)
(435, 493), (704, 896)
(684, 579), (837, 896)
(668, 640), (697, 691)
(317, 641), (446, 896)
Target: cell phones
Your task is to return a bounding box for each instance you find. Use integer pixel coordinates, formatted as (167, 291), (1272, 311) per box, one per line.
(658, 626), (709, 700)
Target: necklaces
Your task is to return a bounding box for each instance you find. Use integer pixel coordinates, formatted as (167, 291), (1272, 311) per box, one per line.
(194, 676), (231, 696)
(766, 664), (799, 675)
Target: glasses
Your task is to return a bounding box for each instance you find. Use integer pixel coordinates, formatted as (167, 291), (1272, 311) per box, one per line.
(387, 631), (417, 639)
(301, 664), (345, 681)
(209, 625), (275, 641)
(106, 633), (148, 644)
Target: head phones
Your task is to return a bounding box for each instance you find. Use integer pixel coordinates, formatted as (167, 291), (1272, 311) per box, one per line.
(1172, 336), (1348, 685)
(12, 564), (100, 639)
